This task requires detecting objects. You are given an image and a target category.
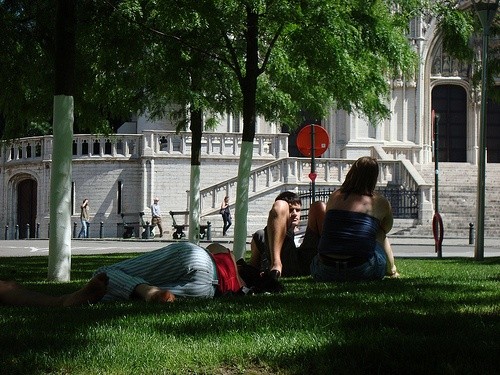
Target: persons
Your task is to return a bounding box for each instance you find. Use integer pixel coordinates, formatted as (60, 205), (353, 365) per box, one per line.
(77, 198), (91, 238)
(309, 154), (400, 282)
(86, 240), (287, 304)
(0, 271), (111, 312)
(149, 198), (165, 238)
(250, 190), (326, 275)
(219, 196), (232, 237)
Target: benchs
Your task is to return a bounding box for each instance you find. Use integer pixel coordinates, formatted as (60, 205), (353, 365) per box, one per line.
(169, 210), (207, 239)
(120, 212), (155, 238)
